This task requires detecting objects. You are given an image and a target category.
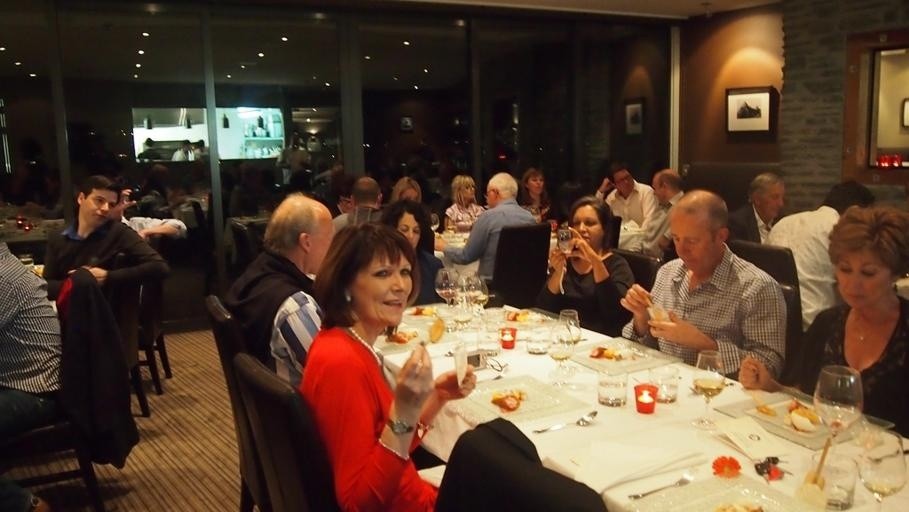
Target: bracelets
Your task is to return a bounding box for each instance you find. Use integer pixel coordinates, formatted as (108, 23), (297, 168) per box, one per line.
(417, 421), (434, 438)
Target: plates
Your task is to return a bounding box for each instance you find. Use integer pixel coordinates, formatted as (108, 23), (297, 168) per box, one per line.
(745, 398), (861, 450)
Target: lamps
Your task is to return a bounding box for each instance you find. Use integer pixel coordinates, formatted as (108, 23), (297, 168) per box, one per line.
(222, 112), (230, 128)
(185, 118), (192, 129)
(144, 117), (154, 130)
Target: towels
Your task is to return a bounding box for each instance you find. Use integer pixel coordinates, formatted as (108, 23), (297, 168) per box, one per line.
(571, 335), (683, 376)
(471, 374), (592, 426)
(379, 319), (458, 355)
(578, 430), (705, 495)
(714, 390), (880, 449)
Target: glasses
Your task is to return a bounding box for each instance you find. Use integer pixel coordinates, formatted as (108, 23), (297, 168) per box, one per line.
(483, 190), (492, 198)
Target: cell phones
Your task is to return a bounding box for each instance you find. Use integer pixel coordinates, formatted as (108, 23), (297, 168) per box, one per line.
(558, 230), (571, 255)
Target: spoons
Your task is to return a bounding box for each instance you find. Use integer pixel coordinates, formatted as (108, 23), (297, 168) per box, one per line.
(532, 411), (597, 434)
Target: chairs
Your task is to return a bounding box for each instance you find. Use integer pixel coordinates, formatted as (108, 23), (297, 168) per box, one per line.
(231, 221), (259, 270)
(731, 239), (810, 354)
(11, 266), (106, 512)
(229, 350), (337, 512)
(435, 430), (607, 511)
(138, 271), (172, 396)
(205, 291), (270, 512)
(481, 222), (554, 312)
(614, 248), (662, 296)
(119, 326), (154, 420)
(185, 227), (253, 296)
(191, 199), (212, 234)
(779, 284), (805, 387)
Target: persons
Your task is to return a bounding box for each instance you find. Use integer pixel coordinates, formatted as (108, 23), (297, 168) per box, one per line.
(766, 177), (874, 334)
(739, 204), (909, 438)
(729, 171), (786, 243)
(103, 176), (188, 243)
(533, 197), (634, 336)
(596, 162), (659, 234)
(522, 169), (568, 230)
(140, 137), (210, 197)
(365, 144), (471, 196)
(330, 174), (356, 217)
(301, 221), (476, 511)
(0, 233), (62, 512)
(435, 172), (537, 306)
(262, 148), (325, 189)
(329, 178), (387, 231)
(620, 189), (786, 383)
(43, 175), (168, 297)
(633, 170), (685, 259)
(385, 201), (448, 305)
(444, 176), (485, 230)
(390, 176), (422, 208)
(230, 191), (441, 470)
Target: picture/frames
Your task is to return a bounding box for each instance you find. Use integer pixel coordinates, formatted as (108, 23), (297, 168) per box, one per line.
(724, 85), (780, 142)
(399, 114), (415, 133)
(621, 98), (645, 140)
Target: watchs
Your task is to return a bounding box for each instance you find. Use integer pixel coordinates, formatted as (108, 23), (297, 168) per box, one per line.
(385, 417), (417, 435)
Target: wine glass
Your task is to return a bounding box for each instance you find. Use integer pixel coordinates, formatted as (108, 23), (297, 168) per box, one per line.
(693, 350), (725, 429)
(19, 254), (34, 273)
(201, 197), (206, 208)
(558, 310), (580, 344)
(814, 366), (863, 470)
(548, 219), (556, 239)
(856, 430), (906, 512)
(430, 213), (439, 239)
(454, 276), (488, 338)
(16, 216), (31, 235)
(813, 454), (859, 511)
(435, 268), (461, 317)
(549, 325), (575, 374)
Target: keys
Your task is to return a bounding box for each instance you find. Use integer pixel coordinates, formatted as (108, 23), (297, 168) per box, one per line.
(755, 453), (794, 483)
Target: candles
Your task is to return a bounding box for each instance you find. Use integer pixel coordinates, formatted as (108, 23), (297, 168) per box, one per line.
(636, 388), (655, 413)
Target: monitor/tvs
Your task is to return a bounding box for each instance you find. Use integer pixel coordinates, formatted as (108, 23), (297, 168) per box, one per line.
(216, 107), (286, 160)
(132, 108), (210, 163)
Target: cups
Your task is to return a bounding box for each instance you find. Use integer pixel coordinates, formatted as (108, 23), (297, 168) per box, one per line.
(527, 326), (550, 354)
(598, 368), (628, 406)
(634, 385), (658, 414)
(649, 366), (679, 403)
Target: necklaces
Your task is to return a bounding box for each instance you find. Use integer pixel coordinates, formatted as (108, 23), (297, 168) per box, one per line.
(348, 326), (386, 368)
(849, 311), (894, 340)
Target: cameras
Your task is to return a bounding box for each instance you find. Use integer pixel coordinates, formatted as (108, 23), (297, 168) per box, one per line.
(466, 350), (488, 370)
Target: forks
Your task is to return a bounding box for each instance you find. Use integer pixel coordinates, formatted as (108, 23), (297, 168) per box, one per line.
(867, 450), (909, 464)
(628, 468), (697, 500)
